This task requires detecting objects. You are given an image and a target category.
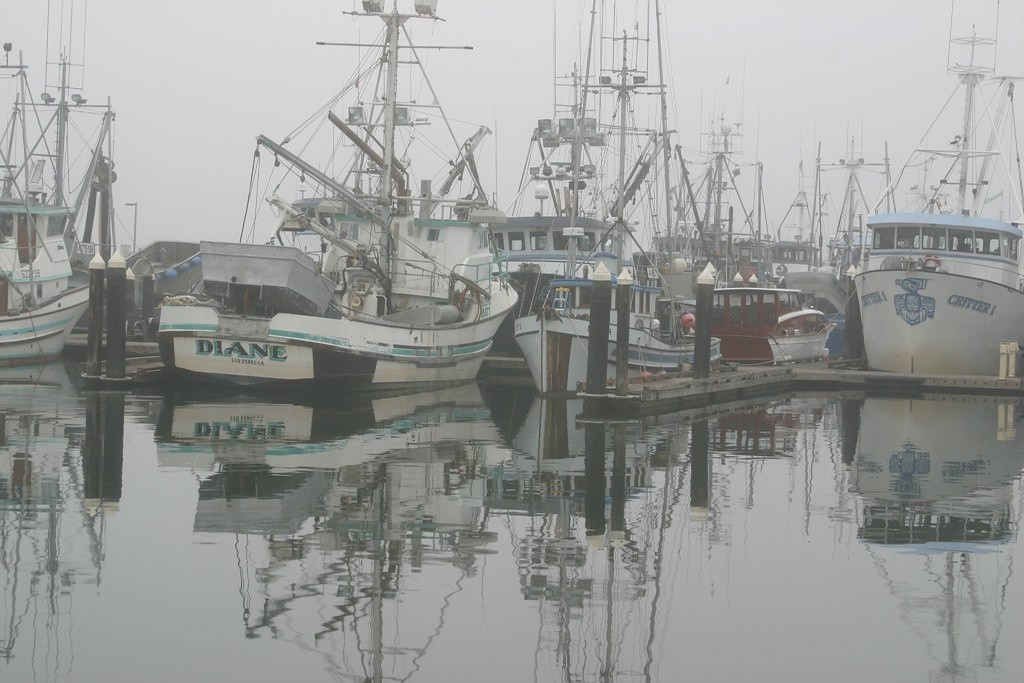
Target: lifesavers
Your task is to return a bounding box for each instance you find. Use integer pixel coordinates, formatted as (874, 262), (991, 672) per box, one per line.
(920, 252), (944, 271)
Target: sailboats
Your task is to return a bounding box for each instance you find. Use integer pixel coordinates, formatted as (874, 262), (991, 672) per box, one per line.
(1, 0), (1024, 402)
